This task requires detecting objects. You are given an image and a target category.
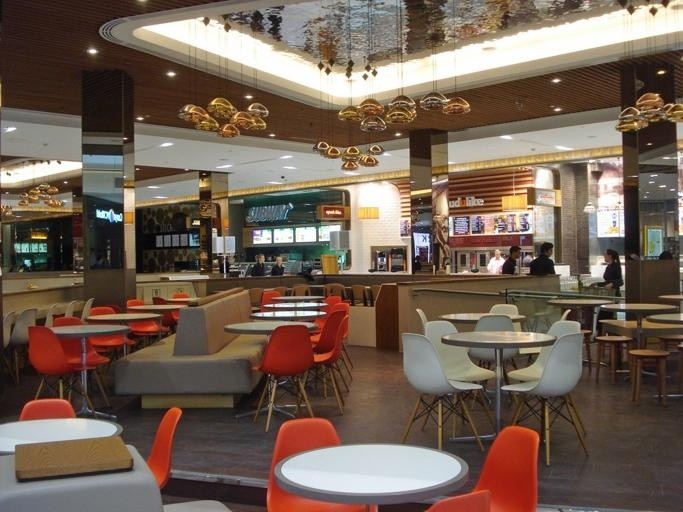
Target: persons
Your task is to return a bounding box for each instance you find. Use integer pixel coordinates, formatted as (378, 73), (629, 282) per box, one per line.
(271, 257), (284, 275)
(251, 253), (265, 276)
(589, 249), (624, 341)
(502, 246), (521, 272)
(530, 243), (555, 274)
(487, 250), (505, 274)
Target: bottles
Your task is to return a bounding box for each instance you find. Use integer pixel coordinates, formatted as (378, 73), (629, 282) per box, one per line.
(564, 276), (570, 289)
(560, 276), (565, 291)
(568, 276), (573, 288)
(573, 276), (578, 288)
(471, 265), (479, 273)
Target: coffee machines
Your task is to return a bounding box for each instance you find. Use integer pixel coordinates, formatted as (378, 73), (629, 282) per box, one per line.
(370, 246), (407, 272)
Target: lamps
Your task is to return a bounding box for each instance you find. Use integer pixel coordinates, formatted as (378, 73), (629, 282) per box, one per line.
(312, 0), (418, 171)
(1, 161), (70, 224)
(357, 185), (380, 219)
(421, 1), (446, 112)
(501, 168), (527, 214)
(441, 0), (470, 116)
(617, 0), (683, 130)
(179, 13), (268, 138)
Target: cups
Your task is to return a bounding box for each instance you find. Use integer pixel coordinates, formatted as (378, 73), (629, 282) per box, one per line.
(446, 265), (451, 275)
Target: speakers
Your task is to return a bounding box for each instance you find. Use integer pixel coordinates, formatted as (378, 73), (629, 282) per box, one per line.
(330, 231), (339, 250)
(225, 236), (235, 254)
(339, 230), (351, 250)
(212, 237), (225, 253)
